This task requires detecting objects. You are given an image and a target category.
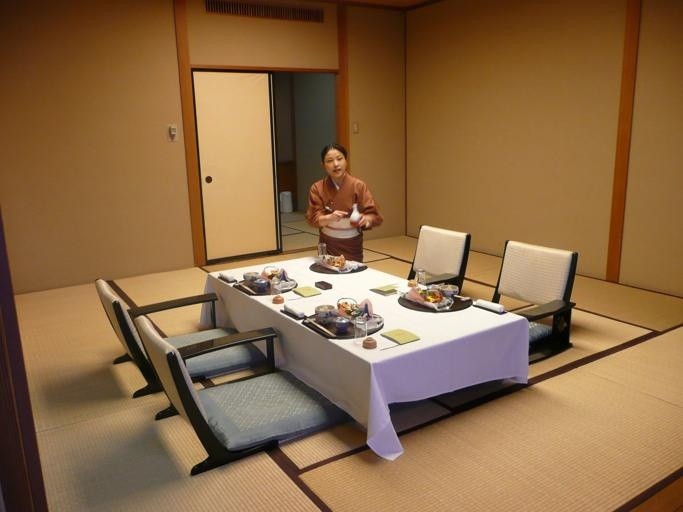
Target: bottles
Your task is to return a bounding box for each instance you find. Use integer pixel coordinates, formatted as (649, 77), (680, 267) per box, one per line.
(349, 203), (363, 229)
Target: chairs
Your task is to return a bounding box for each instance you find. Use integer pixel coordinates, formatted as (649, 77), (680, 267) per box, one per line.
(93, 276), (353, 477)
(406, 224), (580, 367)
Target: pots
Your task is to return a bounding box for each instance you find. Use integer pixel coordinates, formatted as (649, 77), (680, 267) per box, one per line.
(315, 304), (336, 323)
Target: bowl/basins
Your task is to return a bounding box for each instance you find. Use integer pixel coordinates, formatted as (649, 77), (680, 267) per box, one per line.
(361, 337), (377, 349)
(408, 279), (418, 287)
(272, 295), (285, 305)
(439, 284), (459, 297)
(243, 272), (259, 281)
(254, 278), (268, 292)
(336, 317), (349, 333)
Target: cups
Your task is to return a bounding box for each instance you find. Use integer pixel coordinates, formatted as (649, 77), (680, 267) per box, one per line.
(317, 242), (327, 256)
(353, 316), (368, 346)
(270, 279), (281, 299)
(416, 268), (427, 288)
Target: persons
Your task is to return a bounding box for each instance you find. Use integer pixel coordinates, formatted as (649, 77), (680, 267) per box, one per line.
(304, 143), (384, 262)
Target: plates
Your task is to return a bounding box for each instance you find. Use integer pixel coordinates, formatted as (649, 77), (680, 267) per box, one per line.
(302, 308), (384, 339)
(232, 278), (298, 296)
(308, 260), (368, 274)
(398, 289), (473, 313)
